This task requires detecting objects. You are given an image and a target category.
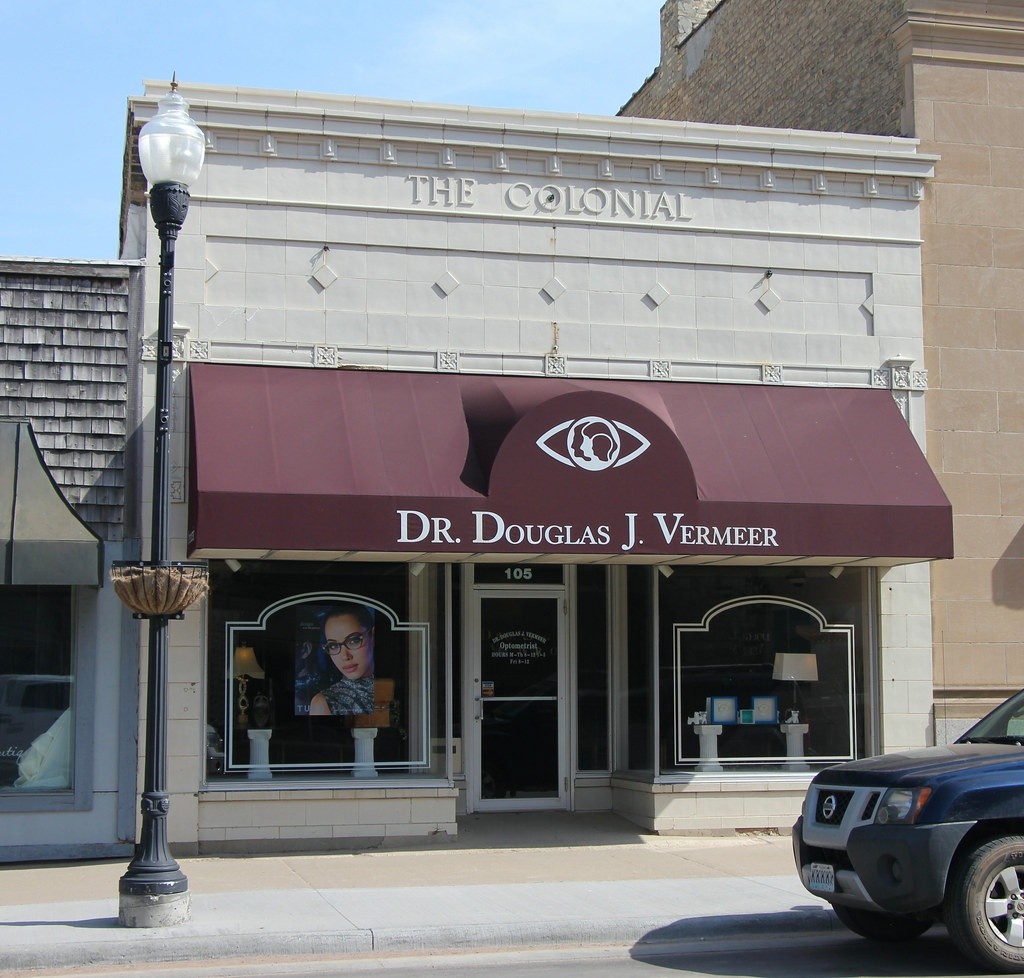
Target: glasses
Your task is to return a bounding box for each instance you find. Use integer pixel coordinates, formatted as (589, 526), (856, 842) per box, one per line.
(322, 624), (374, 655)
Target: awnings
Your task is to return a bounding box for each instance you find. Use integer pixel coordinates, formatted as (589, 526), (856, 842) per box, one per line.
(0, 420), (105, 588)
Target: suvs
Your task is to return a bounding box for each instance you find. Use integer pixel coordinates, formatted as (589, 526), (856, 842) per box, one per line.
(657, 661), (810, 758)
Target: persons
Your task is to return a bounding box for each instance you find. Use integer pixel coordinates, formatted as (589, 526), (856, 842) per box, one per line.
(308, 603), (405, 729)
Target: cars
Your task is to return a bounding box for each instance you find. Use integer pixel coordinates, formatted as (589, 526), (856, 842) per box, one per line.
(790, 685), (1024, 977)
(0, 675), (74, 775)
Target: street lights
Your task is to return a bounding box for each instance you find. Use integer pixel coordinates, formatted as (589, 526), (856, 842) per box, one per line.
(116, 72), (210, 929)
